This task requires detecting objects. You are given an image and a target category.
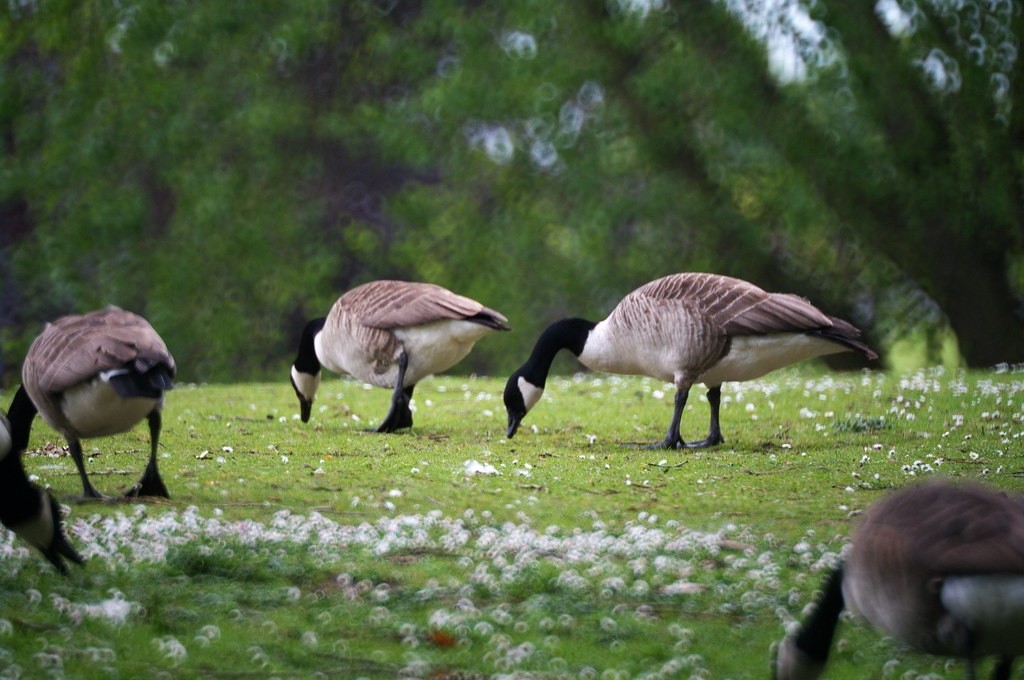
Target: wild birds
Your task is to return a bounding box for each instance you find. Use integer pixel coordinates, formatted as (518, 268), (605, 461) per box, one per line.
(771, 479), (1024, 680)
(290, 279), (512, 433)
(0, 304), (177, 578)
(503, 272), (882, 449)
